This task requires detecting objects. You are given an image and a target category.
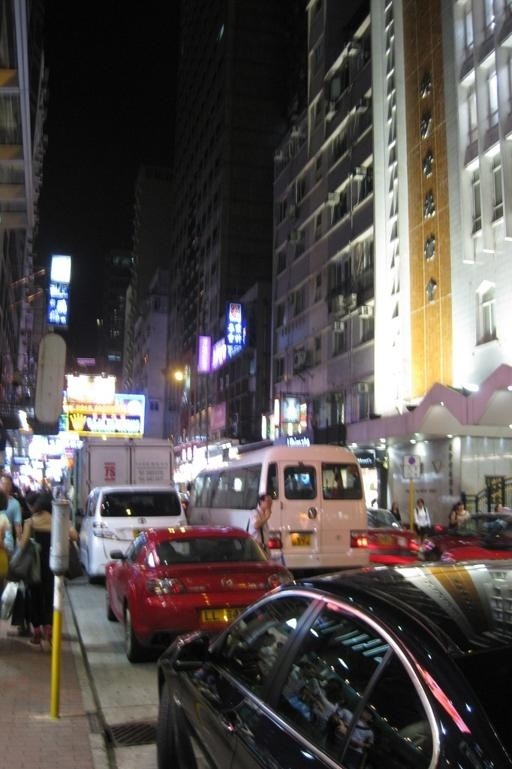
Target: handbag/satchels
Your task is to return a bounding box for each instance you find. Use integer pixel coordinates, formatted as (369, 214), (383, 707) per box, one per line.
(448, 522), (459, 530)
(7, 538), (42, 582)
(1, 579), (20, 620)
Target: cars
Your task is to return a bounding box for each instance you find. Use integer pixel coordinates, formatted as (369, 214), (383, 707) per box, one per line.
(366, 507), (512, 564)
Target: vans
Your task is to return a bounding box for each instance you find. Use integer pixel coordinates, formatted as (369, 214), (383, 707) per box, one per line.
(78, 485), (190, 583)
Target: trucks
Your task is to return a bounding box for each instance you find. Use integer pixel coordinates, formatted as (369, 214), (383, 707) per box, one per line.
(70, 437), (175, 532)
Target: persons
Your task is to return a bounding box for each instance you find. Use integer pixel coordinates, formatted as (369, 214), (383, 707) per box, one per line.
(240, 630), (375, 768)
(450, 501), (470, 528)
(414, 498), (431, 543)
(248, 493), (273, 558)
(493, 503), (503, 512)
(0, 472), (79, 648)
(391, 501), (401, 525)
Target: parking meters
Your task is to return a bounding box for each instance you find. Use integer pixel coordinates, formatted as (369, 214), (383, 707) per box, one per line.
(47, 489), (71, 717)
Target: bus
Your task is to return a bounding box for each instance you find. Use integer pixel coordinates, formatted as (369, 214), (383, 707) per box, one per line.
(189, 444), (371, 571)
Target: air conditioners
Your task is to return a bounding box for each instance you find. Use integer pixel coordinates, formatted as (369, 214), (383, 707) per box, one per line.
(292, 351), (306, 370)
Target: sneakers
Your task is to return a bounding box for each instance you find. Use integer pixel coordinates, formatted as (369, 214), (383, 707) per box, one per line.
(7, 626), (46, 647)
